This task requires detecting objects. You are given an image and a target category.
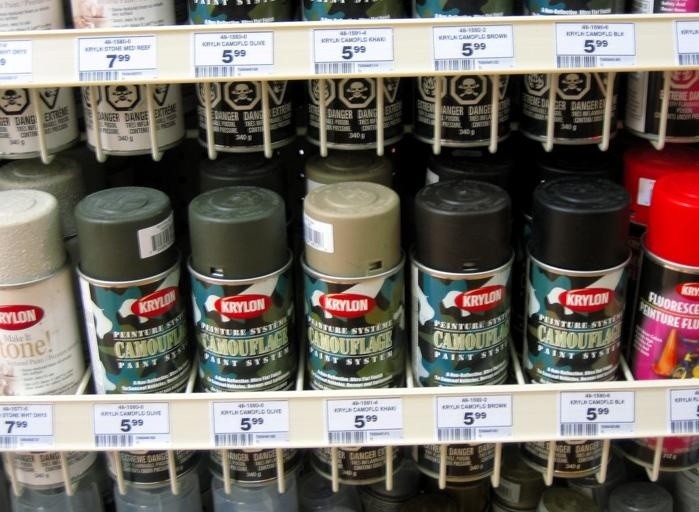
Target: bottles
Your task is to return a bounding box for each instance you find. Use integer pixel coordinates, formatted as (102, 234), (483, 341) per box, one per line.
(1, 158), (695, 399)
(1, 441), (697, 511)
(1, 3), (695, 164)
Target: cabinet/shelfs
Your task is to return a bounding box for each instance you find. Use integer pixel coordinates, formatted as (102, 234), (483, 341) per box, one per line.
(0, 12), (699, 452)
